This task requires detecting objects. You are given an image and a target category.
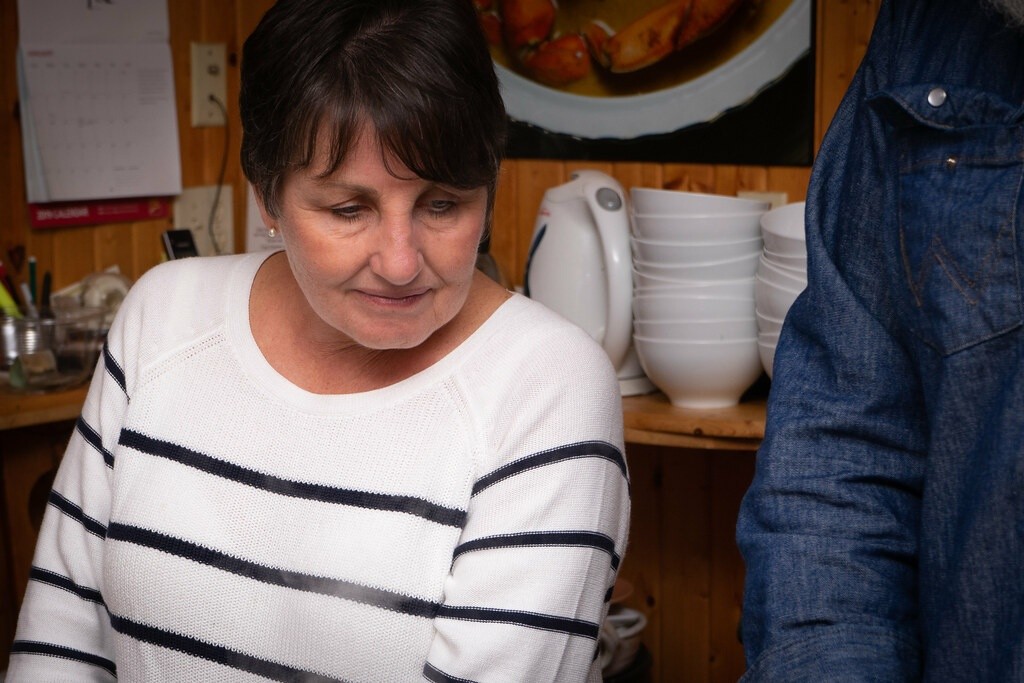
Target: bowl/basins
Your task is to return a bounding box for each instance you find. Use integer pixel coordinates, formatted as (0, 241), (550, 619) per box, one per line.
(2, 304), (104, 393)
(627, 180), (809, 410)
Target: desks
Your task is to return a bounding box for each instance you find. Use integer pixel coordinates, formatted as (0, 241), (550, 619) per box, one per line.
(0, 170), (813, 452)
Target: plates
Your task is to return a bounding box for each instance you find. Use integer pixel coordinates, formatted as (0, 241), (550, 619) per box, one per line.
(490, 1), (814, 141)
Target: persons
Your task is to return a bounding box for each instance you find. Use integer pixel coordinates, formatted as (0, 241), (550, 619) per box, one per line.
(2, 2), (632, 683)
(733, 2), (1024, 683)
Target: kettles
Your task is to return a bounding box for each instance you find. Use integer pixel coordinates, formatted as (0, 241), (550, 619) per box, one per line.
(522, 167), (656, 395)
